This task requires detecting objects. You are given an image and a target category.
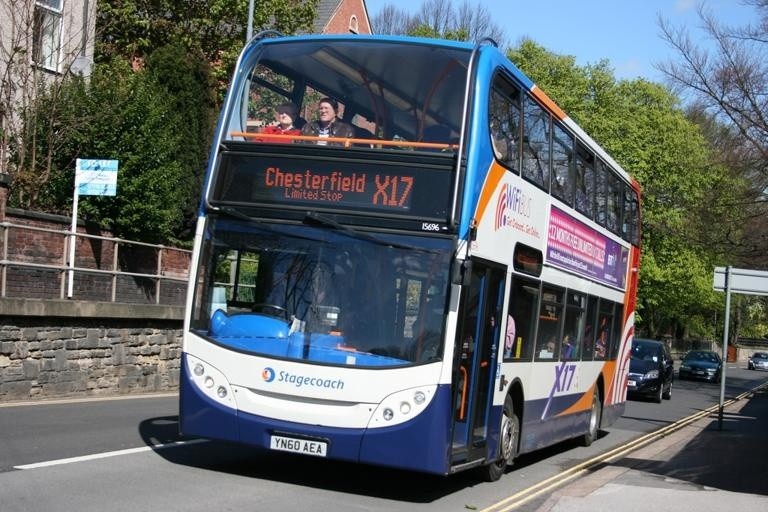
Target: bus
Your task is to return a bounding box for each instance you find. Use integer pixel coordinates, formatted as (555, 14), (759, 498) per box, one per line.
(178, 32), (641, 482)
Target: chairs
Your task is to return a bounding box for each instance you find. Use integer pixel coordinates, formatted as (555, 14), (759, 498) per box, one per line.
(355, 128), (372, 148)
(418, 124), (451, 151)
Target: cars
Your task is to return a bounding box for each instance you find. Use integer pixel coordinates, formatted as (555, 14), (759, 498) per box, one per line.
(748, 352), (767, 371)
(628, 339), (674, 404)
(679, 351), (721, 384)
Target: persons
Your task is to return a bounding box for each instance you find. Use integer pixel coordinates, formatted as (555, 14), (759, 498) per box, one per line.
(249, 101), (302, 143)
(294, 96), (355, 148)
(260, 255), (445, 362)
(488, 312), (609, 358)
